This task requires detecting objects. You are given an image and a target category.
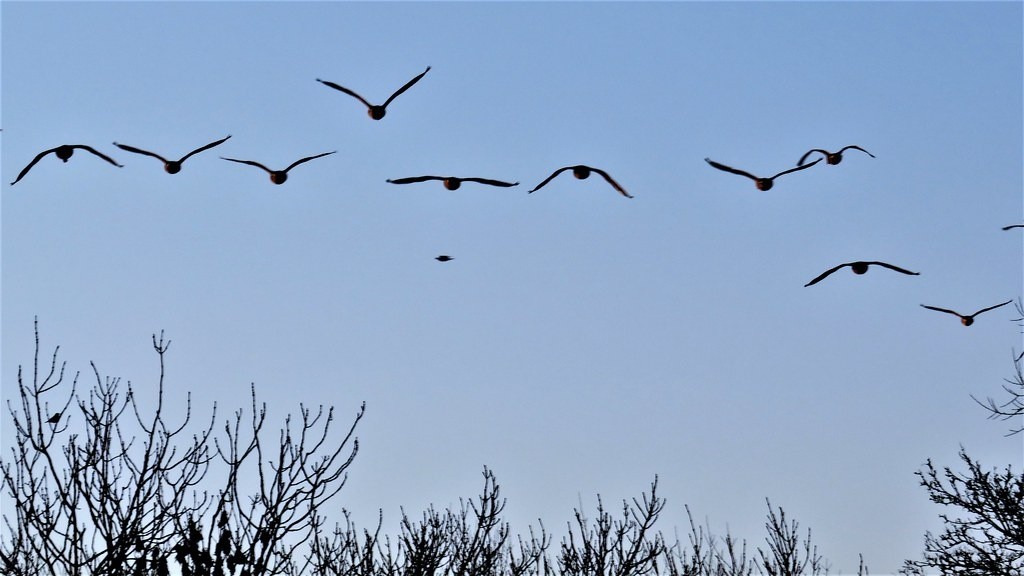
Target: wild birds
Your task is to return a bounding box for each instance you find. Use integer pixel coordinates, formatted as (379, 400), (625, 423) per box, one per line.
(218, 150), (340, 185)
(1000, 224), (1024, 232)
(385, 173), (521, 191)
(803, 261), (921, 288)
(919, 299), (1013, 327)
(526, 164), (636, 201)
(704, 145), (877, 191)
(315, 65), (432, 121)
(111, 134), (234, 174)
(10, 144), (126, 185)
